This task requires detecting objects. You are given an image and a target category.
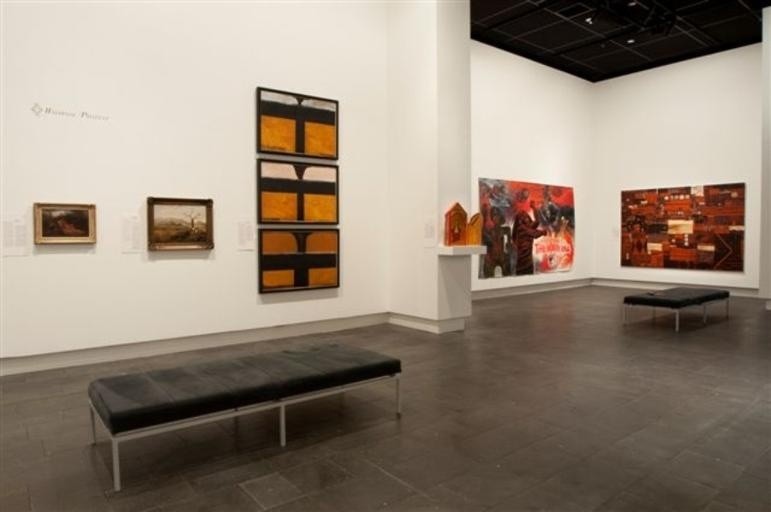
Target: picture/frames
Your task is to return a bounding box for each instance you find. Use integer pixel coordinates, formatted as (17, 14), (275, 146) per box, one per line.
(33, 202), (96, 244)
(147, 196), (214, 251)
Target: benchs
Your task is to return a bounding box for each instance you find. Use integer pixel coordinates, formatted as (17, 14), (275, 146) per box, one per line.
(88, 342), (402, 493)
(623, 287), (729, 332)
